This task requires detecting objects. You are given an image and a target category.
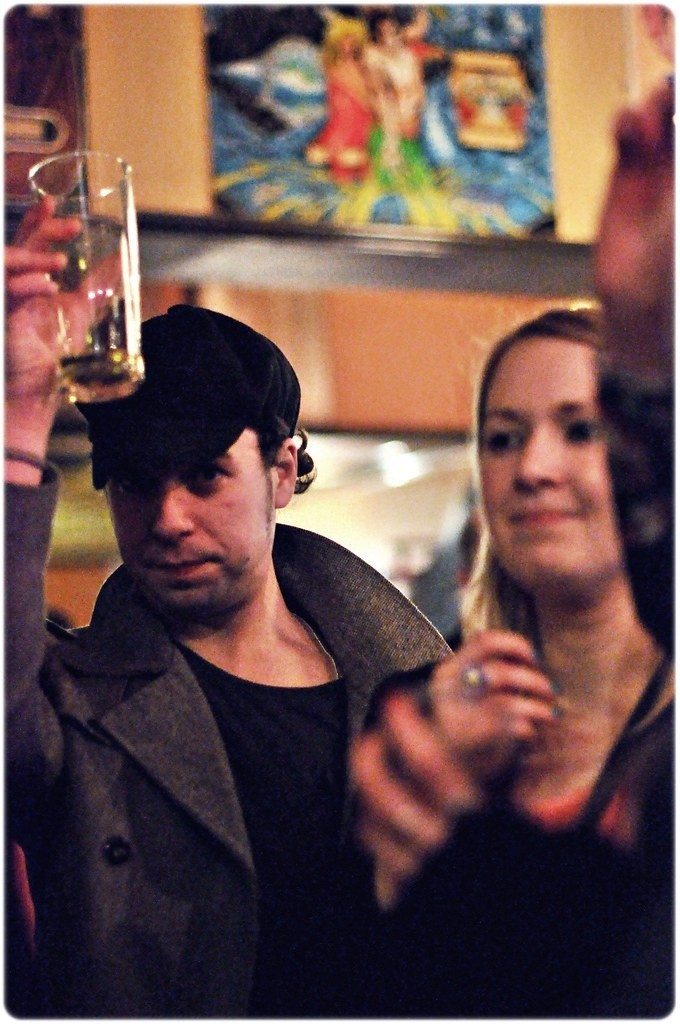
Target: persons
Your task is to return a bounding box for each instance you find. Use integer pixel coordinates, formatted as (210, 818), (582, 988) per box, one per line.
(345, 310), (676, 1022)
(355, 63), (676, 1019)
(4, 196), (456, 1018)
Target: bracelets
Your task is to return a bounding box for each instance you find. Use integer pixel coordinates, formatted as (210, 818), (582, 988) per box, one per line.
(4, 447), (49, 470)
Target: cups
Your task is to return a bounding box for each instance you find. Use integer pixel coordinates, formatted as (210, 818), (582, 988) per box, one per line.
(29, 151), (144, 404)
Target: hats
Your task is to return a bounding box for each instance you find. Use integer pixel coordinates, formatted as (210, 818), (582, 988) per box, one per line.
(76, 304), (300, 490)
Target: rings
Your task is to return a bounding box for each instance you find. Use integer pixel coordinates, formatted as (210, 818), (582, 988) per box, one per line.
(465, 666), (485, 699)
